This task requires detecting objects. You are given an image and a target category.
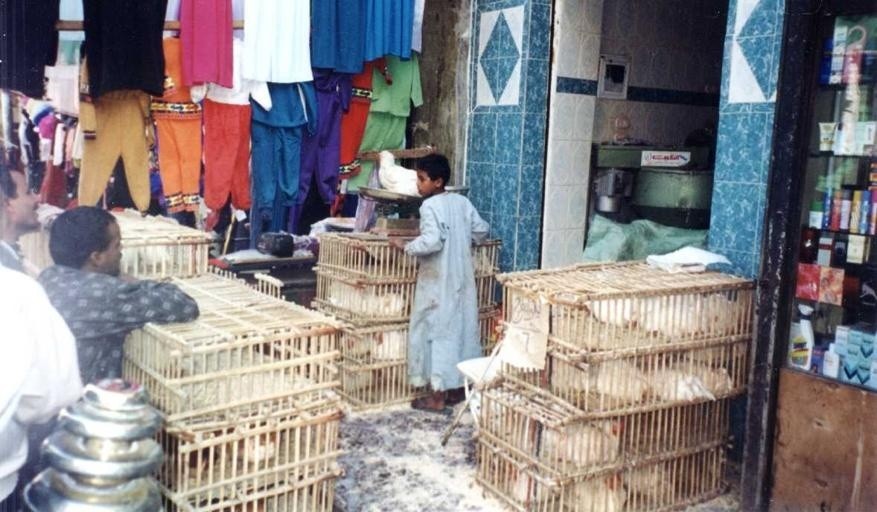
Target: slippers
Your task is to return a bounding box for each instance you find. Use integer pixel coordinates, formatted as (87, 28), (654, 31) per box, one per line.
(446, 389), (468, 404)
(411, 397), (454, 417)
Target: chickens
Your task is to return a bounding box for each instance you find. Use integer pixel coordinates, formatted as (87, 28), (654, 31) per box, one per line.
(511, 465), (562, 503)
(156, 432), (218, 475)
(540, 421), (620, 467)
(212, 427), (280, 463)
(375, 147), (423, 198)
(370, 329), (408, 360)
(334, 365), (375, 394)
(329, 280), (409, 317)
(341, 334), (376, 358)
(561, 474), (626, 512)
(365, 241), (492, 273)
(512, 272), (743, 404)
(619, 470), (674, 502)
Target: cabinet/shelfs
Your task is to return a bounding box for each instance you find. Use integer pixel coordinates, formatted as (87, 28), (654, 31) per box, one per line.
(740, 0), (876, 512)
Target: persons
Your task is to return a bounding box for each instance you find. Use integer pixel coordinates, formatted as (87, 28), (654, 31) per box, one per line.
(2, 162), (46, 273)
(36, 205), (201, 384)
(0, 259), (87, 512)
(383, 152), (494, 412)
